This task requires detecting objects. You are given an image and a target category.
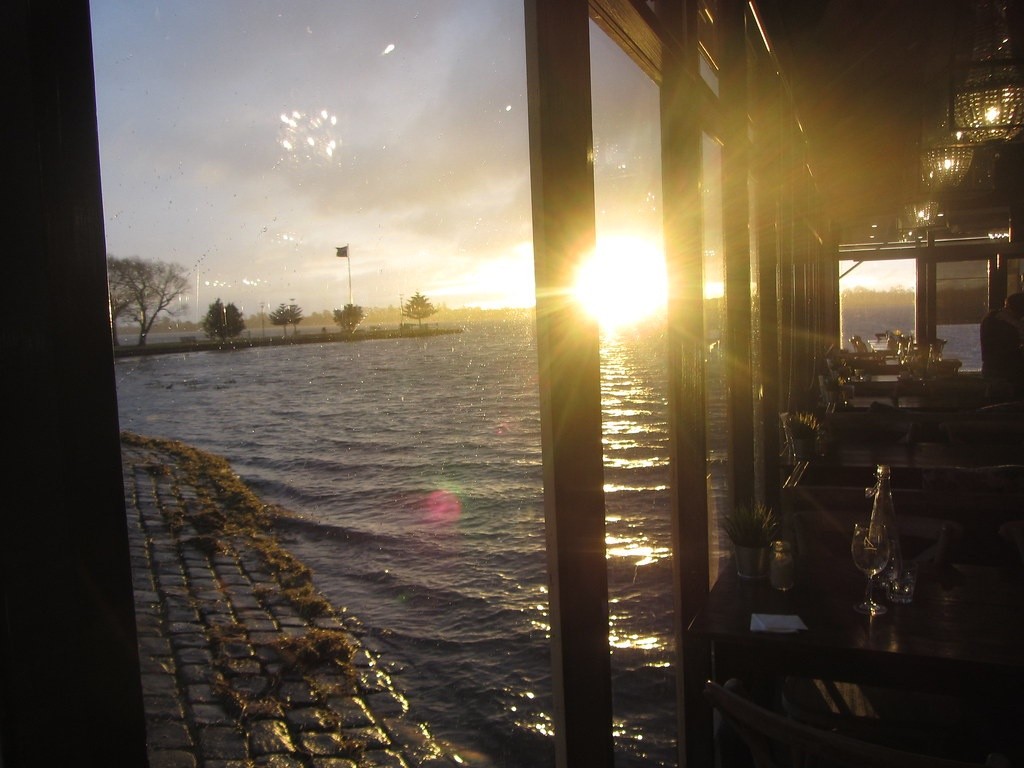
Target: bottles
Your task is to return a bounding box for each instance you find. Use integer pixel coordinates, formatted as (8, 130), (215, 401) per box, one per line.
(869, 465), (903, 587)
(771, 543), (793, 592)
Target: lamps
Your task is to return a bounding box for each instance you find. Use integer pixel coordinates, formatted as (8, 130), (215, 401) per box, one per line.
(896, 0), (1024, 237)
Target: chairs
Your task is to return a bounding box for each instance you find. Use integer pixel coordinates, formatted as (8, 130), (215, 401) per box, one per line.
(705, 680), (982, 768)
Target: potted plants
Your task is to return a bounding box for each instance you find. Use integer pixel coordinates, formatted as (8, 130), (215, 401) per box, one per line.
(824, 371), (847, 405)
(718, 497), (781, 581)
(780, 410), (825, 460)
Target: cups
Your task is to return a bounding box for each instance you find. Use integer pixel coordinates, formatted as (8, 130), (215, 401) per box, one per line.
(886, 560), (917, 605)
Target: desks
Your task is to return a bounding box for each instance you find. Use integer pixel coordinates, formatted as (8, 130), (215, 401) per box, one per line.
(688, 345), (1024, 768)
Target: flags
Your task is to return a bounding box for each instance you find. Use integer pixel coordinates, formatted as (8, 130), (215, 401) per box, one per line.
(336, 244), (350, 257)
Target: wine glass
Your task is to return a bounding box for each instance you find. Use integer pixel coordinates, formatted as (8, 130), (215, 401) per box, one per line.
(929, 343), (944, 380)
(854, 359), (865, 385)
(851, 522), (888, 615)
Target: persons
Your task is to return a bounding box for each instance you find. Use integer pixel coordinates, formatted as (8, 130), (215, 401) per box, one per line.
(981, 293), (1024, 407)
